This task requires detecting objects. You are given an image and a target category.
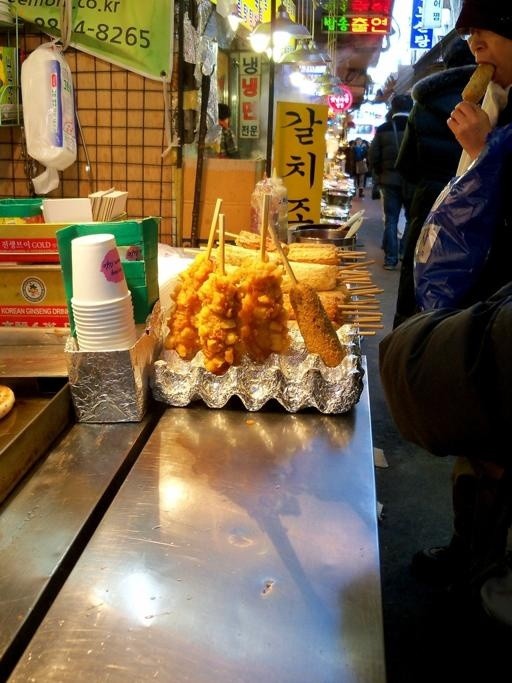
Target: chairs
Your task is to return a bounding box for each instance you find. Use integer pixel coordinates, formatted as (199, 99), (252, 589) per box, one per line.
(384, 254), (406, 271)
(412, 544), (446, 567)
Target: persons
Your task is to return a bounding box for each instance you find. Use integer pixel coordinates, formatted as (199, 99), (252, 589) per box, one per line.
(392, 36), (485, 329)
(218, 103), (240, 159)
(366, 95), (414, 270)
(380, 285), (509, 595)
(447, 0), (512, 300)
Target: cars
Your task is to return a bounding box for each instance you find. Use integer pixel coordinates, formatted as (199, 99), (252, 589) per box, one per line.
(70, 232), (139, 354)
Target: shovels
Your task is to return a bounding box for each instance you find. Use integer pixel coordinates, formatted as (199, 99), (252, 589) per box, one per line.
(0, 384), (16, 420)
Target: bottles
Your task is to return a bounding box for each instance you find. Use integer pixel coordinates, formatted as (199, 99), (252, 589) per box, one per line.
(454, 0), (512, 42)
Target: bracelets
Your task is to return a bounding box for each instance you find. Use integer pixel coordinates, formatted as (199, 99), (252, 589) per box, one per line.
(295, 224), (357, 262)
